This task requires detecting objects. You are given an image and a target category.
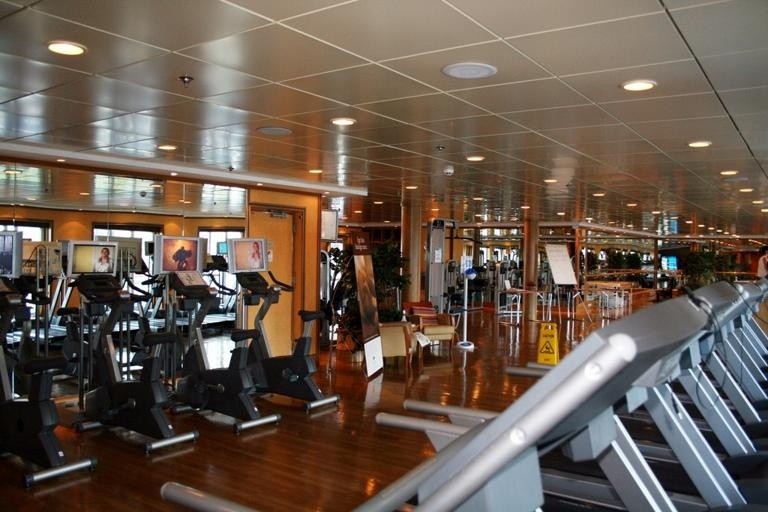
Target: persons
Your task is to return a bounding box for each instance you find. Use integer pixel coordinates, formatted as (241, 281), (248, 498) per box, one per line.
(93, 247), (114, 273)
(250, 241), (262, 267)
(756, 245), (767, 281)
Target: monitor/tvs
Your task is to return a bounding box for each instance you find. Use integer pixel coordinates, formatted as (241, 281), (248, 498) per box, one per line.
(144, 241), (153, 256)
(227, 238), (269, 274)
(0, 231), (22, 280)
(217, 242), (227, 254)
(21, 242), (63, 276)
(95, 235), (143, 273)
(65, 240), (120, 278)
(153, 234), (208, 275)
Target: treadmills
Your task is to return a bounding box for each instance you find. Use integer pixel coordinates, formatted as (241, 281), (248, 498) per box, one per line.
(160, 294), (714, 512)
(504, 276), (767, 442)
(375, 272), (768, 512)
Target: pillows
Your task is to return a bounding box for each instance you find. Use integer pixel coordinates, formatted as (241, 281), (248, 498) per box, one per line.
(411, 306), (438, 327)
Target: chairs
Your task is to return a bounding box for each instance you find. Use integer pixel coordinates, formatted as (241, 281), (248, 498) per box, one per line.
(400, 298), (459, 371)
(379, 323), (414, 381)
